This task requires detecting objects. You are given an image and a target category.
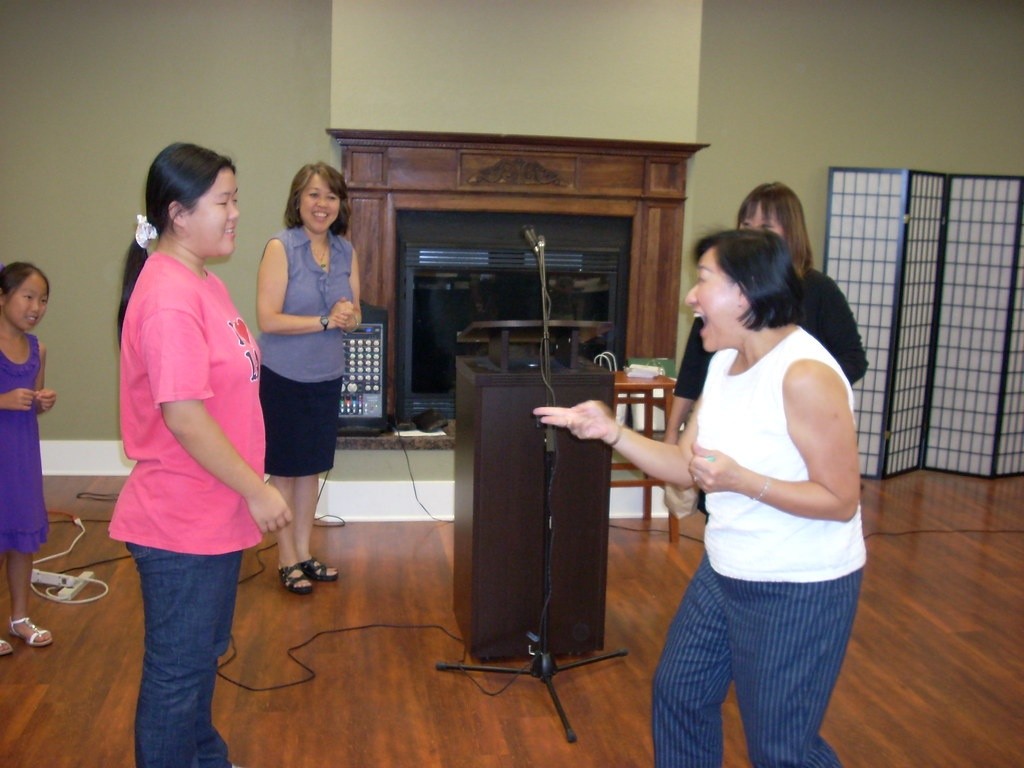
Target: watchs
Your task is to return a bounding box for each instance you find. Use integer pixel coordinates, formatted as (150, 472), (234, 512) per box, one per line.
(320, 314), (329, 331)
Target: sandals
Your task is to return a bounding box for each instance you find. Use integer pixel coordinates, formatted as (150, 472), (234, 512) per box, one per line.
(9, 616), (53, 646)
(279, 562), (313, 595)
(299, 557), (339, 581)
(0, 640), (13, 655)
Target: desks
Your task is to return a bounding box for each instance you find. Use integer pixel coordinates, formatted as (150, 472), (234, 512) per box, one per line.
(609, 371), (679, 543)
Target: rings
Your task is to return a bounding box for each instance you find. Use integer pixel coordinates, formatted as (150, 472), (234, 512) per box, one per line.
(692, 476), (699, 482)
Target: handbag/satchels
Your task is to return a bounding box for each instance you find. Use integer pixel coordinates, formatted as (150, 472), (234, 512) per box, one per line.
(664, 483), (699, 519)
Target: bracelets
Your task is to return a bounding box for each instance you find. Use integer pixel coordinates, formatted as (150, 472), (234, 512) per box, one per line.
(346, 316), (358, 333)
(753, 476), (769, 501)
(609, 425), (621, 446)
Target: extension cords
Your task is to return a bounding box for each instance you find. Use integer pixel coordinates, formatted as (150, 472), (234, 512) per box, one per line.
(58, 571), (95, 600)
(29, 568), (75, 588)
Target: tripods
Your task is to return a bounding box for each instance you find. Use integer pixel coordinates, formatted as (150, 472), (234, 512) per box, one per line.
(436, 233), (628, 742)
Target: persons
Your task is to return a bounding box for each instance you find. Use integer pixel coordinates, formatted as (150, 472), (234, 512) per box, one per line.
(0, 260), (58, 657)
(107, 142), (292, 768)
(533, 231), (869, 768)
(666, 184), (868, 524)
(256, 160), (362, 597)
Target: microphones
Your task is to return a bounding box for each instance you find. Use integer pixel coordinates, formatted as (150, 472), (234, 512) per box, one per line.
(519, 223), (540, 263)
